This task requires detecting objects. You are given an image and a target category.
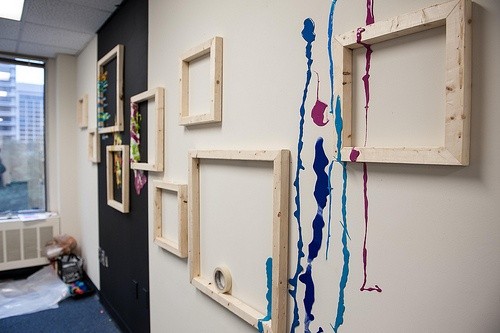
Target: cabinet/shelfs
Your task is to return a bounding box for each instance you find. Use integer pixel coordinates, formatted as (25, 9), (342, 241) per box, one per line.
(0, 216), (59, 272)
